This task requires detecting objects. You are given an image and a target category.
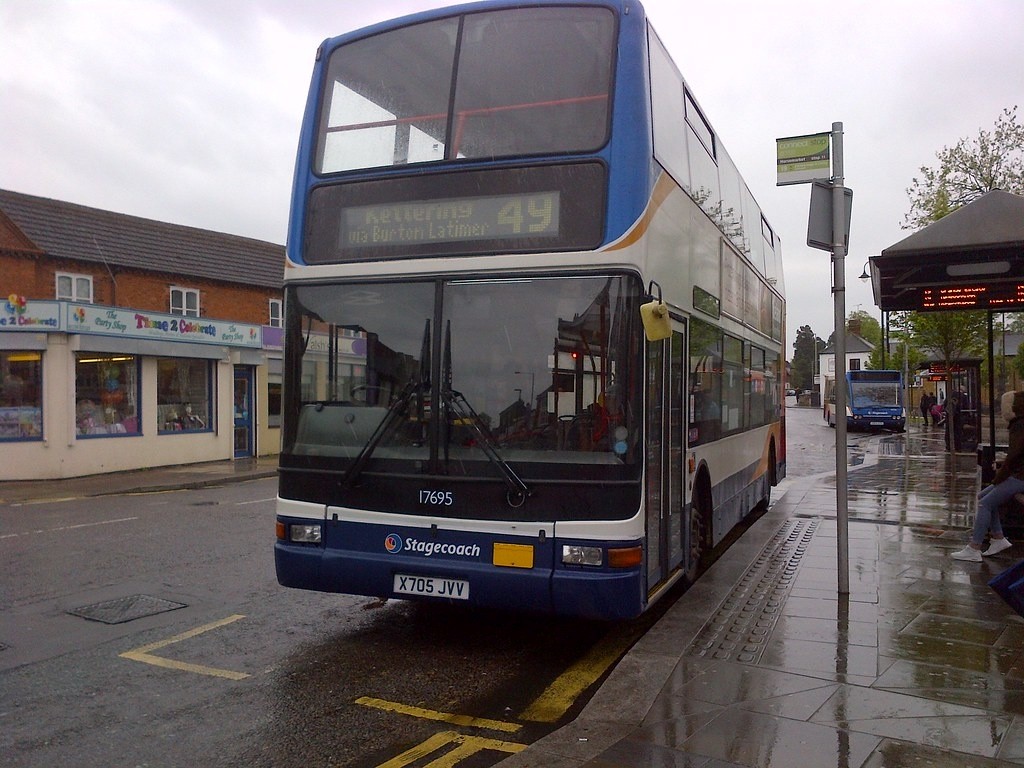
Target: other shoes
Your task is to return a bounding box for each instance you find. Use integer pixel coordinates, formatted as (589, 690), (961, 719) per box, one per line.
(923, 424), (928, 426)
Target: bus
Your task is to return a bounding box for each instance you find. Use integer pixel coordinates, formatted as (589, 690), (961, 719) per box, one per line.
(822, 369), (906, 432)
(272, 0), (790, 617)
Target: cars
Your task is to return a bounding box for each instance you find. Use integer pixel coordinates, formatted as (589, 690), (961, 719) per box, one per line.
(786, 390), (795, 396)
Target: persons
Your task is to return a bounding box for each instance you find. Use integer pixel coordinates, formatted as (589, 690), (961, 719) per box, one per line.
(795, 388), (802, 403)
(943, 385), (968, 453)
(939, 388), (944, 404)
(931, 404), (945, 425)
(950, 391), (1024, 562)
(920, 390), (929, 426)
(928, 392), (937, 413)
(593, 385), (628, 431)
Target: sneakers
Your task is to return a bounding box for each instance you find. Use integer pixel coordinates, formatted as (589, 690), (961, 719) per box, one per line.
(981, 537), (1012, 555)
(951, 544), (983, 562)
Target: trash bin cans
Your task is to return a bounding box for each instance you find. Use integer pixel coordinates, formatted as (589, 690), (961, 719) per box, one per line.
(976, 444), (1009, 516)
